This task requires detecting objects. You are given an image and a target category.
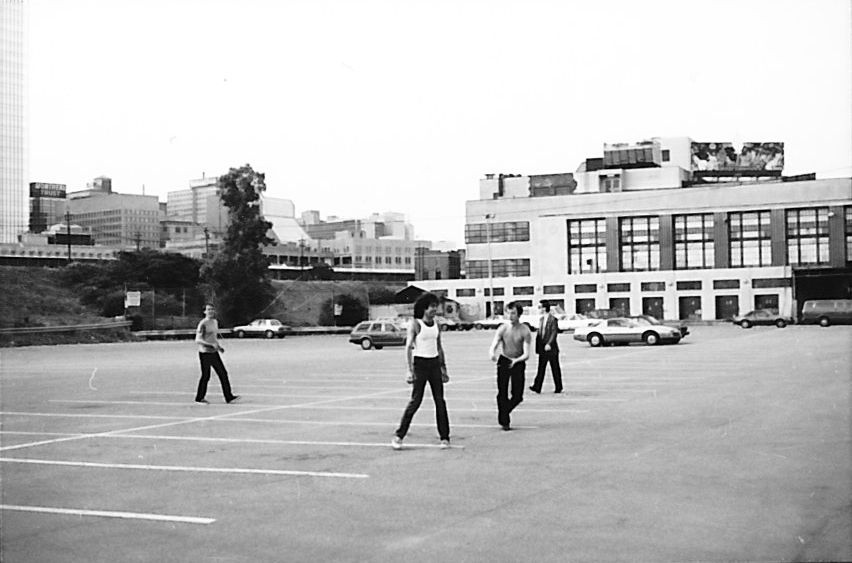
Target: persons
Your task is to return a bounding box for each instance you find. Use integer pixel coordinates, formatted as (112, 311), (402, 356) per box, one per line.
(691, 143), (784, 169)
(528, 300), (563, 394)
(195, 303), (241, 405)
(391, 293), (450, 449)
(490, 302), (530, 430)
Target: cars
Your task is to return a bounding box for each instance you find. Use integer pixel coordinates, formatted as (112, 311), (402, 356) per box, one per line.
(573, 317), (682, 348)
(233, 318), (293, 339)
(348, 320), (407, 350)
(435, 305), (623, 333)
(625, 314), (690, 338)
(733, 310), (794, 329)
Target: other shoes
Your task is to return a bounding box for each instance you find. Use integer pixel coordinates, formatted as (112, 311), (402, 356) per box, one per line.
(529, 386), (540, 393)
(502, 423), (510, 431)
(441, 439), (449, 449)
(196, 398), (209, 404)
(229, 395), (240, 404)
(392, 435), (402, 450)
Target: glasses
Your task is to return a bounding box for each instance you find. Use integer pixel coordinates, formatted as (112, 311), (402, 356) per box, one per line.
(538, 305), (542, 308)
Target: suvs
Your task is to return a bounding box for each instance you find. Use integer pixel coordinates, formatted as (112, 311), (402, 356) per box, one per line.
(801, 299), (852, 327)
